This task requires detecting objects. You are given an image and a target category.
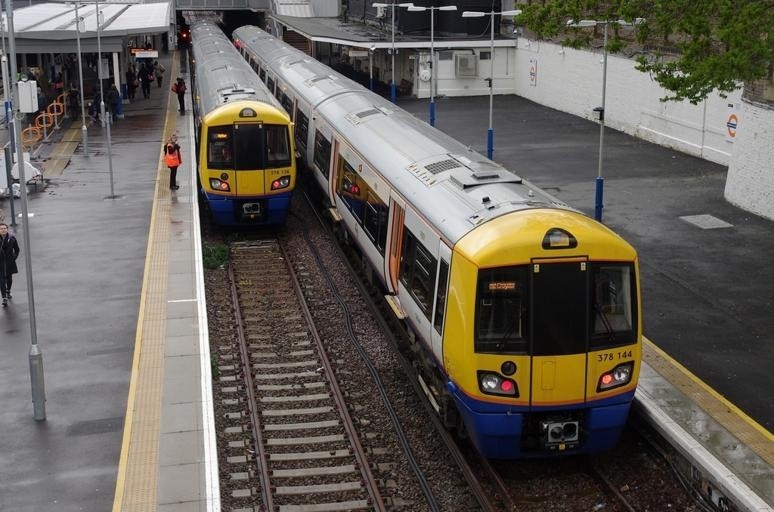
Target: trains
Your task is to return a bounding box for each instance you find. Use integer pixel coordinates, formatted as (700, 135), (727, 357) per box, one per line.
(184, 10), (297, 234)
(231, 25), (642, 466)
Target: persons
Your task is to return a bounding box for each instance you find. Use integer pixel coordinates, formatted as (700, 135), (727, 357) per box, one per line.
(125, 67), (139, 103)
(153, 60), (166, 89)
(173, 77), (187, 116)
(146, 58), (156, 77)
(146, 42), (152, 51)
(0, 221), (21, 309)
(66, 81), (83, 122)
(163, 134), (183, 190)
(86, 101), (99, 123)
(105, 82), (123, 126)
(136, 62), (154, 99)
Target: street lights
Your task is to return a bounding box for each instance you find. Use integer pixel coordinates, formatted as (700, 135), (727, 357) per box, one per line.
(406, 4), (458, 129)
(567, 16), (649, 222)
(461, 9), (524, 161)
(371, 3), (415, 106)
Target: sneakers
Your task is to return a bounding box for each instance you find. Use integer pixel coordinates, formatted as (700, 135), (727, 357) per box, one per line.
(170, 186), (177, 189)
(1, 298), (7, 307)
(174, 185), (178, 188)
(5, 288), (11, 299)
